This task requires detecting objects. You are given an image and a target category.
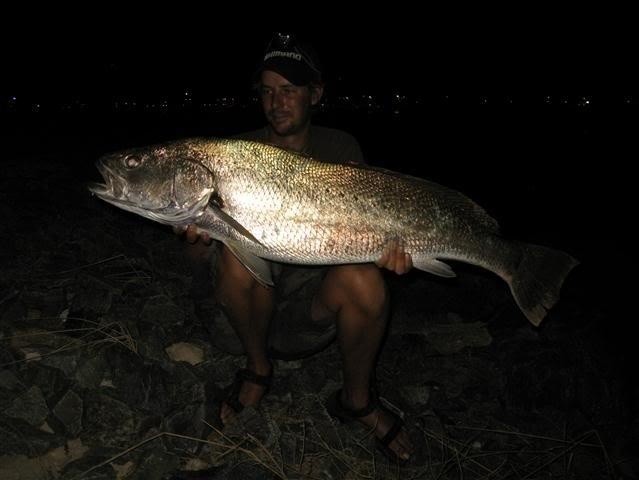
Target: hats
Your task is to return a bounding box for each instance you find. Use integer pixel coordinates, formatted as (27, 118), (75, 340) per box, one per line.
(258, 33), (322, 86)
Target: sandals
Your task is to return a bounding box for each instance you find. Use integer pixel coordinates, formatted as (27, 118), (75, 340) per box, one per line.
(324, 387), (418, 466)
(215, 365), (273, 430)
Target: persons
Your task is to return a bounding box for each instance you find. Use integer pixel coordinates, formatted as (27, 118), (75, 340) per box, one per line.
(169, 30), (418, 467)
